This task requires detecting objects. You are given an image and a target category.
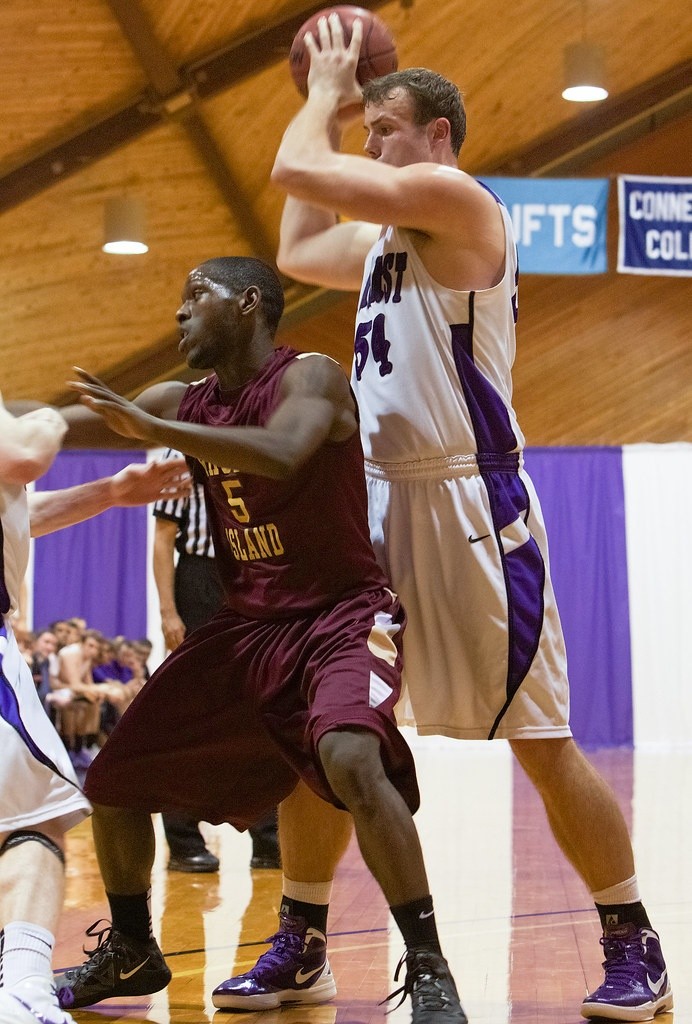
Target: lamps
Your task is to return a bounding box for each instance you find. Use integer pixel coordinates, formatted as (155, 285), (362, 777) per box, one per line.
(103, 152), (149, 255)
(563, 0), (610, 101)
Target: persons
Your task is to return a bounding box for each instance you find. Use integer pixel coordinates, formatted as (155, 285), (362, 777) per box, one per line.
(0, 255), (470, 1024)
(151, 447), (282, 872)
(0, 391), (191, 1024)
(211, 14), (673, 1024)
(10, 613), (155, 767)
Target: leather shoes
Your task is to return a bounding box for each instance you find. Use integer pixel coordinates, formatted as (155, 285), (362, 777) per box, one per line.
(166, 843), (220, 873)
(250, 827), (280, 869)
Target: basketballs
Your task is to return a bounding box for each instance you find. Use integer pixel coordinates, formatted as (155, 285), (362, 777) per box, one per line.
(291, 6), (398, 119)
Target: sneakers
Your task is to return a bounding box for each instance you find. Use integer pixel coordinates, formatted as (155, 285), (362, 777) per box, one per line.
(54, 918), (173, 1008)
(2, 976), (79, 1024)
(210, 917), (338, 1010)
(378, 937), (469, 1024)
(71, 750), (94, 768)
(83, 746), (102, 759)
(579, 929), (674, 1022)
